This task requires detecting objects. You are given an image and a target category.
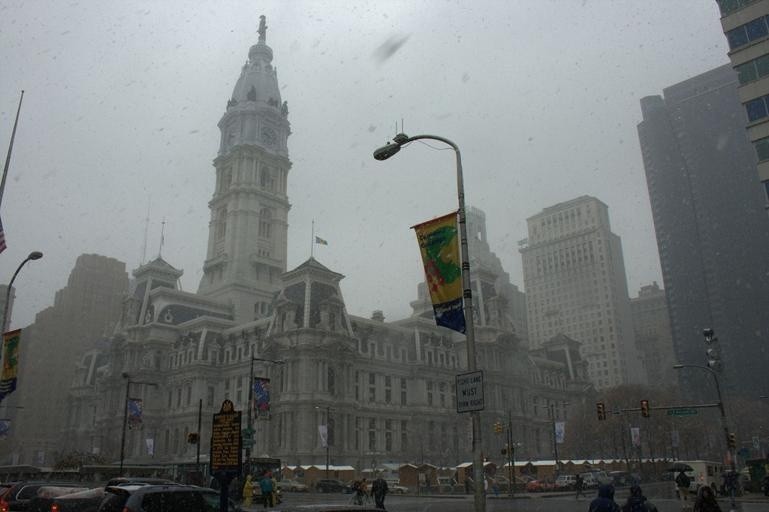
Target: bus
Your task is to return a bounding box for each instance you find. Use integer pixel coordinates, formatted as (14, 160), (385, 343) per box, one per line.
(0, 459), (283, 500)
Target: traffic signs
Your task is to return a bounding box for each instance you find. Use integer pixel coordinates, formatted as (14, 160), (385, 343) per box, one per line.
(667, 409), (698, 416)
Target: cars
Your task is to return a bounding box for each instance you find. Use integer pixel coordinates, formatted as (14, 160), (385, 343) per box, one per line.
(0, 476), (245, 512)
(276, 473), (411, 496)
(423, 464), (644, 492)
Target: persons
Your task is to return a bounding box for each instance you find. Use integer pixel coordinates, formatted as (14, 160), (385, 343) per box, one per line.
(352, 471), (389, 510)
(268, 472), (277, 506)
(243, 475), (256, 508)
(722, 471), (741, 509)
(621, 484), (658, 512)
(675, 472), (691, 509)
(575, 474), (586, 500)
(587, 484), (621, 511)
(692, 485), (722, 512)
(260, 473), (273, 508)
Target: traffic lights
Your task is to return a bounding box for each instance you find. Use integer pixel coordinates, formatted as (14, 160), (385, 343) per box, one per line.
(492, 418), (501, 435)
(639, 399), (650, 417)
(729, 432), (737, 449)
(596, 401), (607, 421)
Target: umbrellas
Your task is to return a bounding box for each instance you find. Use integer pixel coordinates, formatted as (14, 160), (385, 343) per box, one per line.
(666, 463), (694, 473)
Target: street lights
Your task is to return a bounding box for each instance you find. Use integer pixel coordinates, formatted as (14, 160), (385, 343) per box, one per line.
(243, 355), (288, 480)
(671, 362), (734, 461)
(0, 250), (44, 334)
(370, 130), (486, 511)
(118, 378), (161, 475)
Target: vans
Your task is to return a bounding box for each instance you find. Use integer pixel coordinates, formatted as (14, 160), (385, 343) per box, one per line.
(670, 454), (726, 494)
